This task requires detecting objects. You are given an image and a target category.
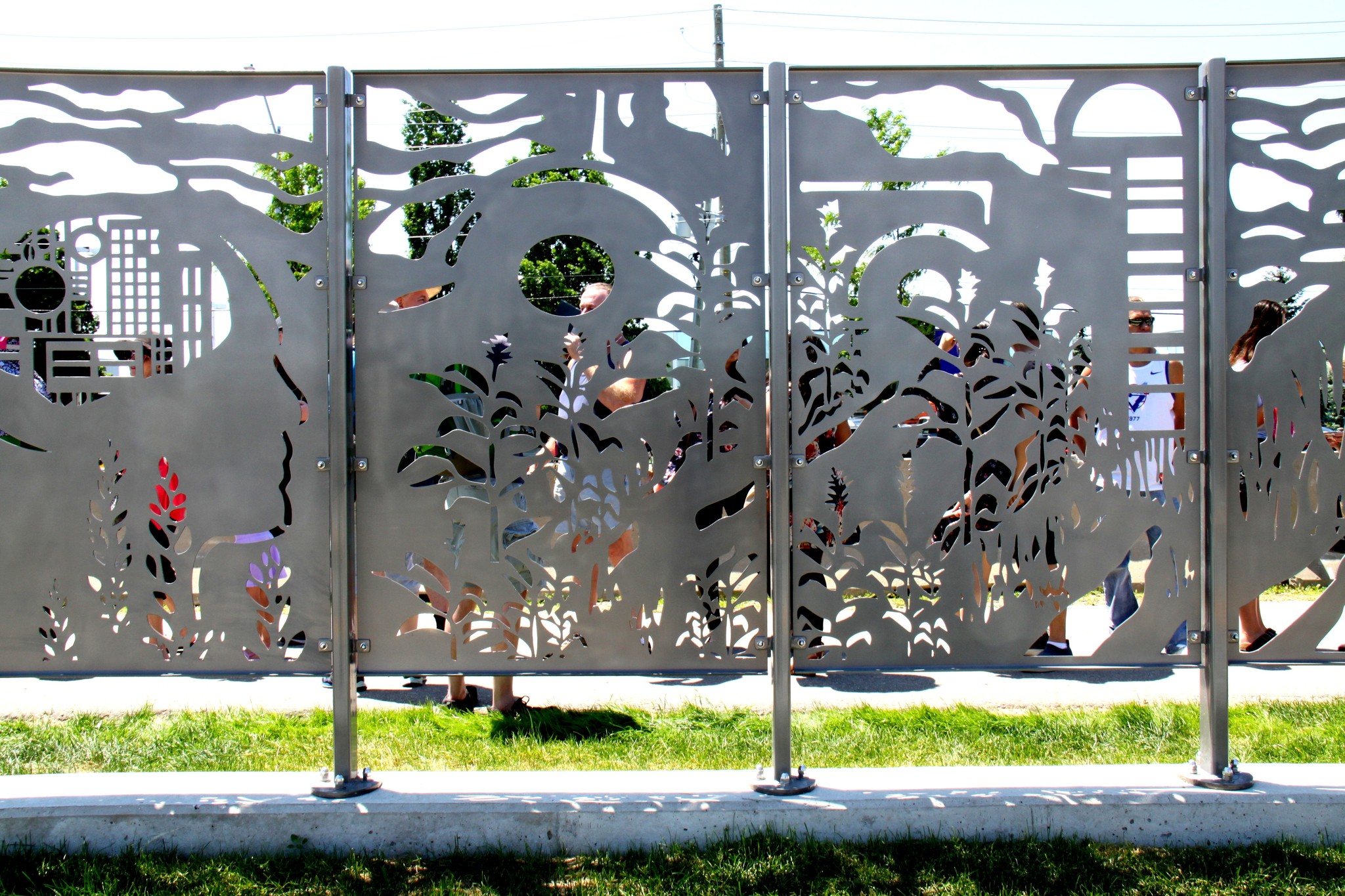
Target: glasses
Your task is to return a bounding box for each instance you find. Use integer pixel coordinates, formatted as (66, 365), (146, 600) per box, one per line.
(1128, 316), (1154, 326)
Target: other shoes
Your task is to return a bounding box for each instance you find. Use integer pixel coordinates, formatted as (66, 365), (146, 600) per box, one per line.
(488, 695), (529, 715)
(1239, 628), (1280, 652)
(403, 675), (427, 687)
(1018, 638), (1074, 674)
(1023, 628), (1050, 655)
(440, 685), (477, 711)
(543, 632), (588, 661)
(789, 641), (826, 678)
(320, 677), (367, 692)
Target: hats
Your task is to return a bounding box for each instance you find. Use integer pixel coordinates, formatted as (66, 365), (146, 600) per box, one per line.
(112, 331), (173, 375)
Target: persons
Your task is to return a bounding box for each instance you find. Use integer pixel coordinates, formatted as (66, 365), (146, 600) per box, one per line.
(1227, 295), (1344, 653)
(0, 329), (58, 641)
(98, 333), (199, 661)
(259, 313), (324, 650)
(1065, 299), (1195, 658)
(218, 531), (288, 664)
(426, 354), (544, 715)
(679, 299), (1072, 675)
(531, 277), (656, 662)
(322, 281), (446, 692)
